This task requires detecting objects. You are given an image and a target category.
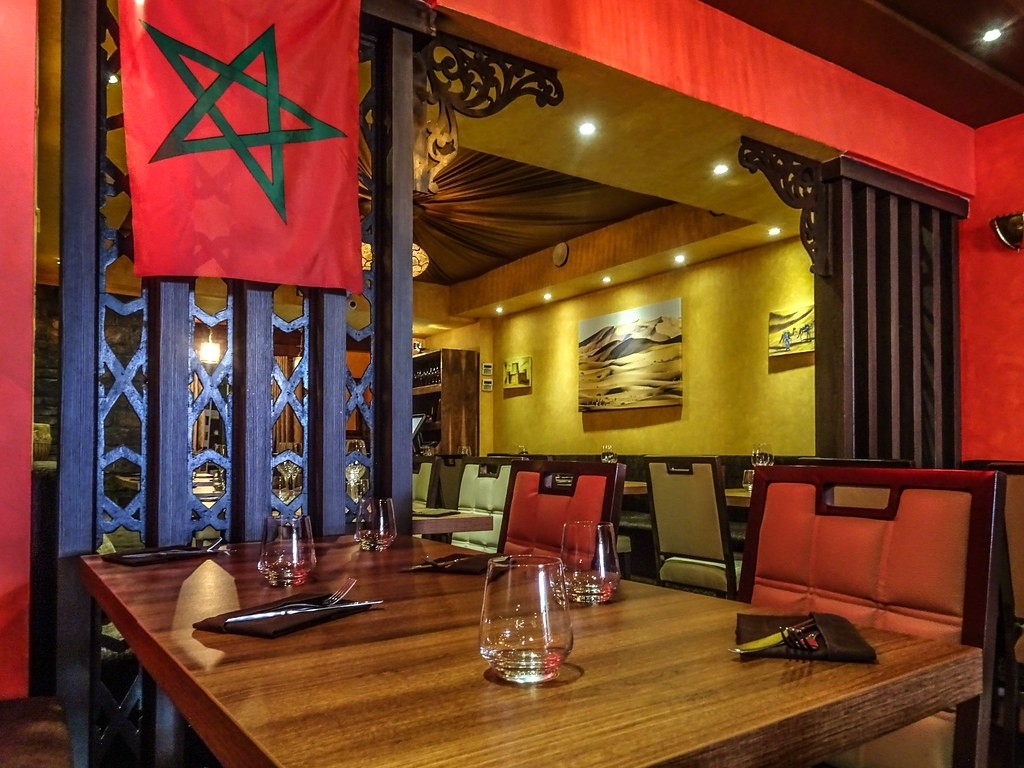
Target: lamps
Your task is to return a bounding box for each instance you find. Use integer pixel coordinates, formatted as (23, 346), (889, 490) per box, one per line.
(199, 324), (221, 364)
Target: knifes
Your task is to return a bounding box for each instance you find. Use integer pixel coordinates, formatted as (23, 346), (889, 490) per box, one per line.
(226, 600), (382, 622)
(400, 557), (468, 569)
(728, 619), (814, 654)
(122, 549), (230, 558)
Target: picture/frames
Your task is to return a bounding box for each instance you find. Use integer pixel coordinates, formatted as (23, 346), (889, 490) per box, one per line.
(502, 356), (531, 388)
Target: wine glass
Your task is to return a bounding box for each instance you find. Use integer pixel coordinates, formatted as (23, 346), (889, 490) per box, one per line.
(276, 443), (302, 496)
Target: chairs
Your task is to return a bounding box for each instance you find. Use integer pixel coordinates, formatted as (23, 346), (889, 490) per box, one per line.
(496, 461), (626, 570)
(644, 455), (743, 601)
(412, 455), (441, 538)
(433, 456), (471, 511)
(450, 457), (528, 556)
(736, 462), (1006, 767)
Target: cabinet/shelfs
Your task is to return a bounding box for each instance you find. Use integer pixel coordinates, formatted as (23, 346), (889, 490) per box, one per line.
(412, 349), (480, 455)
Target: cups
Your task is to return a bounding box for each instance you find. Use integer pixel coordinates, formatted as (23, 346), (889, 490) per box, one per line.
(478, 554), (573, 682)
(458, 446), (471, 456)
(751, 442), (774, 467)
(213, 472), (226, 492)
(345, 440), (369, 501)
(257, 516), (315, 586)
(602, 445), (618, 463)
(214, 445), (227, 474)
(742, 470), (754, 490)
(560, 520), (619, 602)
(355, 498), (397, 551)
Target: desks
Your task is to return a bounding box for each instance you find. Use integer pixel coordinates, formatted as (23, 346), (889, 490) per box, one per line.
(77, 530), (980, 767)
(112, 472), (225, 498)
(411, 506), (492, 545)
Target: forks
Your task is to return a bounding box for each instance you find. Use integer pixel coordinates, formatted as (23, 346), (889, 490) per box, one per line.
(171, 536), (222, 551)
(264, 577), (357, 612)
(780, 624), (821, 650)
(421, 555), (452, 569)
